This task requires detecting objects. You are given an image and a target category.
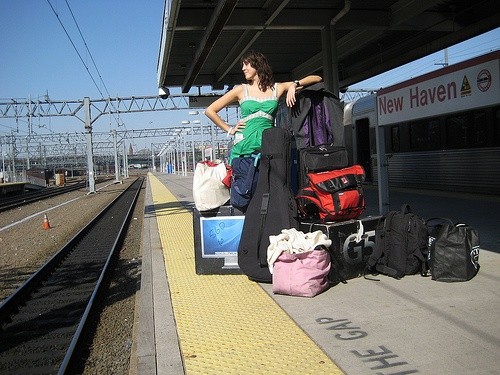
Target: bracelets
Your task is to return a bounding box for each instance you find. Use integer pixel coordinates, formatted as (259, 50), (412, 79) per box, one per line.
(227, 128), (232, 136)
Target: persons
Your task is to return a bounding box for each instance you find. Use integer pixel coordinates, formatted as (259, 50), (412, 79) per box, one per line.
(205, 49), (322, 164)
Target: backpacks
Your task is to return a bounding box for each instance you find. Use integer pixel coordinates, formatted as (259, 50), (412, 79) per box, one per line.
(362, 204), (429, 279)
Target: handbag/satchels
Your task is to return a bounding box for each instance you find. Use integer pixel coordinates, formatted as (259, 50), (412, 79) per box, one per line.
(429, 222), (480, 283)
(296, 143), (349, 186)
(271, 244), (333, 298)
(192, 158), (232, 212)
(230, 149), (262, 214)
(295, 164), (366, 223)
(425, 216), (456, 243)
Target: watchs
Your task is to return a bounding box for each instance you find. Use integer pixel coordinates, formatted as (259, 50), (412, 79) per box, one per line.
(294, 80), (300, 87)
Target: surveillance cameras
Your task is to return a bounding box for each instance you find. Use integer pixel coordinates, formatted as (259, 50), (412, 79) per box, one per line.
(159, 87), (171, 100)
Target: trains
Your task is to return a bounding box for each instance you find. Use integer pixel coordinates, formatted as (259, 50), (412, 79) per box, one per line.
(344, 90), (500, 204)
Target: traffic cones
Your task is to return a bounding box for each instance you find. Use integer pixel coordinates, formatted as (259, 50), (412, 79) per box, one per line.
(44, 213), (50, 230)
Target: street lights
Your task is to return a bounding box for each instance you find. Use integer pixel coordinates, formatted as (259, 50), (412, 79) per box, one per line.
(188, 110), (206, 160)
(157, 127), (191, 177)
(181, 120), (201, 173)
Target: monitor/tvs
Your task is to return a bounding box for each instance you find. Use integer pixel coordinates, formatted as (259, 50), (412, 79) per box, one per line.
(200, 216), (246, 269)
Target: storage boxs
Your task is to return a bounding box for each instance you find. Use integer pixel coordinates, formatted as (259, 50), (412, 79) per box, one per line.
(301, 215), (385, 282)
